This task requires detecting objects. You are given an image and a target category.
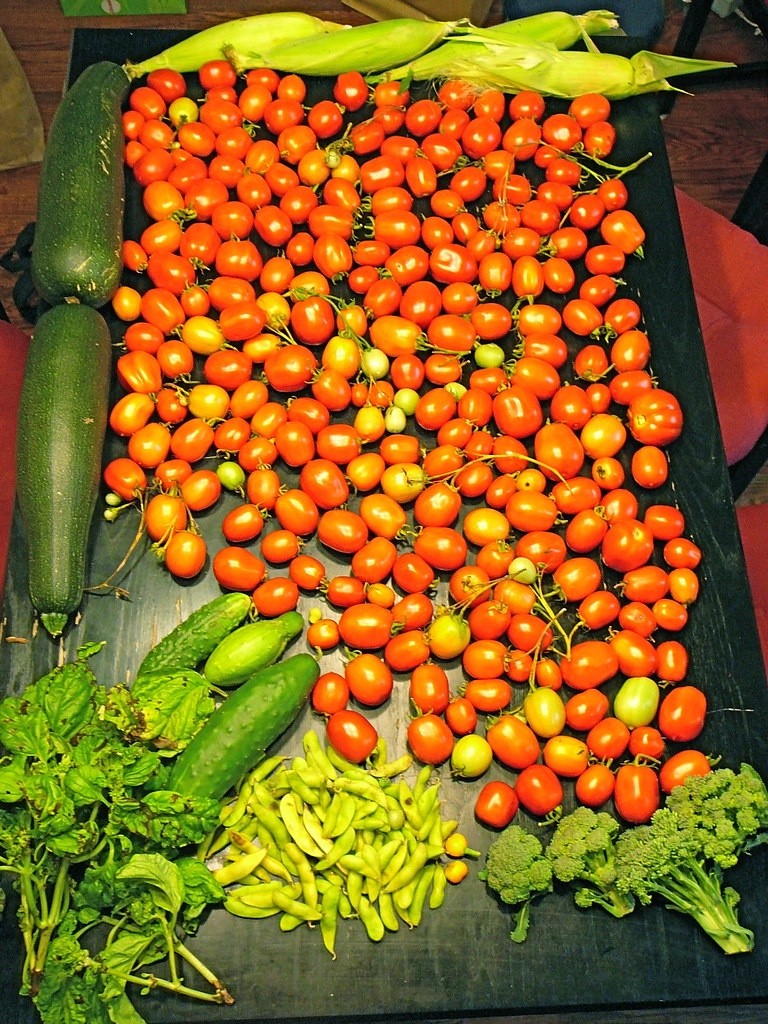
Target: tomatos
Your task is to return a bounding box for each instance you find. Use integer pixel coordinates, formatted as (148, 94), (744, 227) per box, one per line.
(103, 59), (722, 825)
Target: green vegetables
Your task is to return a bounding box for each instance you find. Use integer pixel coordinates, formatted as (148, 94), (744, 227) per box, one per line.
(0, 636), (236, 1024)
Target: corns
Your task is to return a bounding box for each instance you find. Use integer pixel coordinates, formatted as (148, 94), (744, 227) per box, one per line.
(122, 11), (672, 99)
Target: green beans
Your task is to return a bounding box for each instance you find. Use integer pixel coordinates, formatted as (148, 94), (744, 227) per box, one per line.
(195, 730), (457, 957)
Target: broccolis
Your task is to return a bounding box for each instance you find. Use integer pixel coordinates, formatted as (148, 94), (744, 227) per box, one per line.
(478, 767), (768, 955)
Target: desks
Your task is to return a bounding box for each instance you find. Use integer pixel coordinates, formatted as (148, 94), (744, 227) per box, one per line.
(1, 28), (767, 1023)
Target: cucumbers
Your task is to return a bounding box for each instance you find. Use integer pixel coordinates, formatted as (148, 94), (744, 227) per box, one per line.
(204, 611), (305, 687)
(136, 592), (251, 678)
(167, 653), (320, 802)
